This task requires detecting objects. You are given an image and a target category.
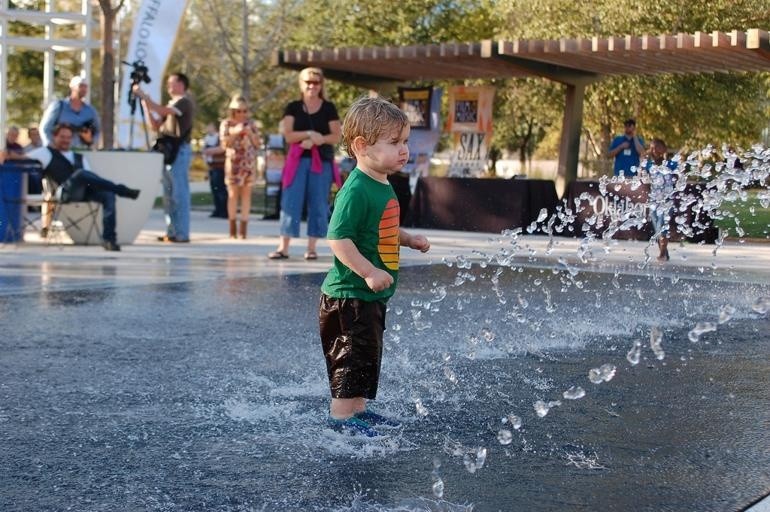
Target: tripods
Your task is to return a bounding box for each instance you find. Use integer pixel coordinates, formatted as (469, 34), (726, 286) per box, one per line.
(127, 83), (152, 152)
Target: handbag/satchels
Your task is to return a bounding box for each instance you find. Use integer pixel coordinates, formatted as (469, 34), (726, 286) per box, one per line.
(152, 133), (179, 164)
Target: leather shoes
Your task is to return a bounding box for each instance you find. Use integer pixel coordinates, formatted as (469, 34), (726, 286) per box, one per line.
(105, 241), (119, 251)
(129, 189), (140, 199)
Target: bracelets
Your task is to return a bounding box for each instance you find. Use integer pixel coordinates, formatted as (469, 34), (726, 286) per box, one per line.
(305, 129), (313, 139)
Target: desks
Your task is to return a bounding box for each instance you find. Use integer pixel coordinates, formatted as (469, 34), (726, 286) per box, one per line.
(389, 174), (411, 225)
(566, 178), (718, 243)
(415, 176), (556, 232)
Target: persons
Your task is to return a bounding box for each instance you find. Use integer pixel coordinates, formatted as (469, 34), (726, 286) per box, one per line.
(265, 67), (342, 260)
(201, 119), (308, 222)
(218, 93), (261, 240)
(5, 126), (24, 152)
(317, 96), (430, 436)
(22, 127), (42, 212)
(37, 75), (101, 238)
(638, 137), (679, 261)
(132, 72), (196, 242)
(606, 118), (770, 187)
(0, 122), (140, 250)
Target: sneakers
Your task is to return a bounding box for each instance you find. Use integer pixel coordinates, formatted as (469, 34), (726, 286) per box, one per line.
(354, 410), (402, 430)
(324, 415), (382, 436)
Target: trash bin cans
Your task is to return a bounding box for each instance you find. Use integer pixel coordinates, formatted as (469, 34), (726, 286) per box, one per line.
(0, 164), (28, 242)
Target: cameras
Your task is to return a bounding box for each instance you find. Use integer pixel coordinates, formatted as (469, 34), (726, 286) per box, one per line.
(130, 60), (151, 83)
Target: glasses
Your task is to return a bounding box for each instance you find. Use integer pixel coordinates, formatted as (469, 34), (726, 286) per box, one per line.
(303, 80), (320, 85)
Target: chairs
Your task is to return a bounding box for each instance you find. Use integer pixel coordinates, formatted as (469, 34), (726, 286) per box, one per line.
(45, 168), (103, 248)
(1, 158), (58, 249)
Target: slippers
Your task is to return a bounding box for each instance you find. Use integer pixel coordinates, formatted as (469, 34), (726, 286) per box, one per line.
(269, 251), (289, 259)
(304, 252), (317, 260)
